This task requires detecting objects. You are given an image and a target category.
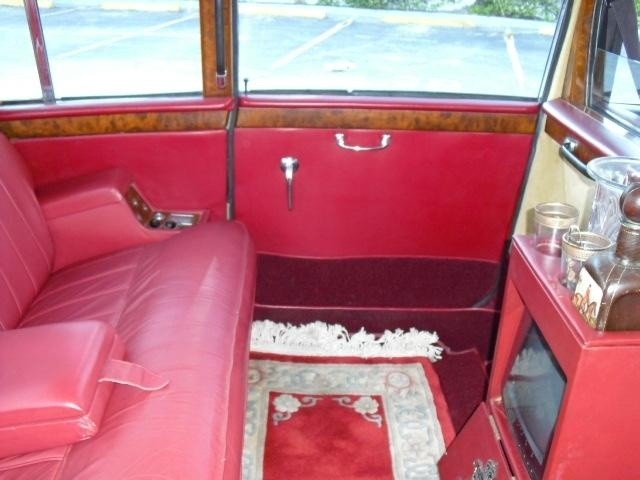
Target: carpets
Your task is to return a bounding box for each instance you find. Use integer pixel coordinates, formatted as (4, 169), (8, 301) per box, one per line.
(242, 320), (457, 480)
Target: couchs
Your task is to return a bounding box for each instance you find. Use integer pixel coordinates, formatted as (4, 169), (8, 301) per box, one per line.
(0, 128), (258, 480)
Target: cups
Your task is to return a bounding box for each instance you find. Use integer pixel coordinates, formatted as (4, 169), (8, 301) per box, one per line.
(532, 200), (614, 291)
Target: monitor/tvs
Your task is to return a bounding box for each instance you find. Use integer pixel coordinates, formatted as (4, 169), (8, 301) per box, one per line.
(502, 321), (568, 479)
